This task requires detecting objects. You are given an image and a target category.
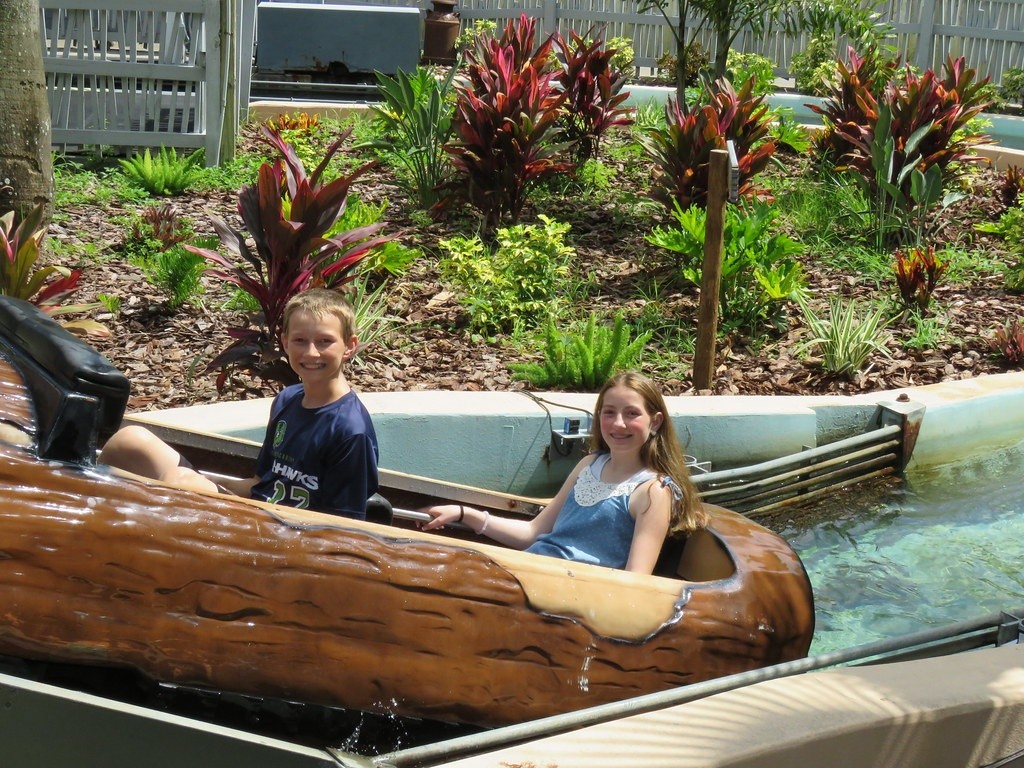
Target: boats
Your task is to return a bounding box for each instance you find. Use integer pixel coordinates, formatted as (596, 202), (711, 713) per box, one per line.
(0, 296), (816, 729)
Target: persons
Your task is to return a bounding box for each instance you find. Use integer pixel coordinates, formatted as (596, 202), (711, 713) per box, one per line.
(414, 371), (711, 575)
(96, 287), (379, 521)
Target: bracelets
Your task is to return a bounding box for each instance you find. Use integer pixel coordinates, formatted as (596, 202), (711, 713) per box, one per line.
(475, 510), (489, 535)
(454, 505), (464, 523)
